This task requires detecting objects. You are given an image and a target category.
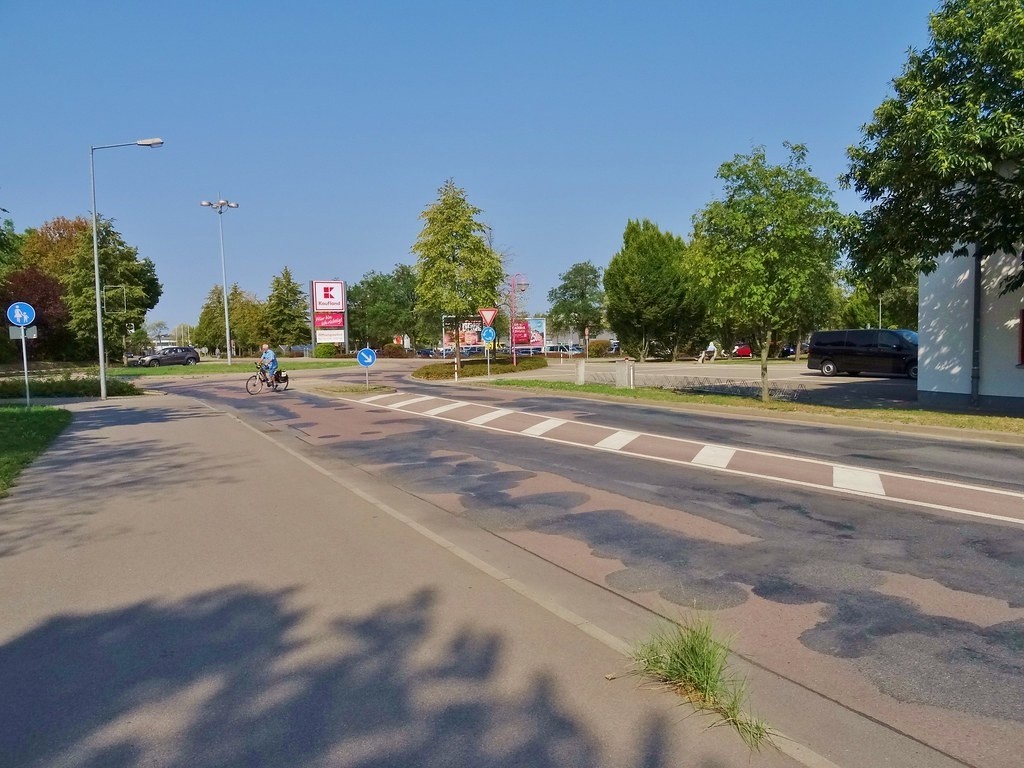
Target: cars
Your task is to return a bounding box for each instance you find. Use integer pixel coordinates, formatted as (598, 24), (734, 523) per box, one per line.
(138, 346), (200, 367)
(516, 350), (538, 355)
(721, 341), (809, 357)
(417, 346), (485, 358)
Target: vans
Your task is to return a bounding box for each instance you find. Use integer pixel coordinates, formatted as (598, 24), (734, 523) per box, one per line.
(809, 329), (918, 380)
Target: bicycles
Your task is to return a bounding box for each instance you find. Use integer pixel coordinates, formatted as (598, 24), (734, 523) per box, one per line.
(246, 362), (289, 395)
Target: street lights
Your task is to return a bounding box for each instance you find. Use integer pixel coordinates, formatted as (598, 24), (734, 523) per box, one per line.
(200, 192), (240, 366)
(510, 271), (530, 366)
(89, 138), (164, 401)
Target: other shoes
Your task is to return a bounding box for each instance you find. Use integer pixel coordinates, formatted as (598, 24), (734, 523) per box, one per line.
(262, 378), (268, 382)
(273, 384), (278, 390)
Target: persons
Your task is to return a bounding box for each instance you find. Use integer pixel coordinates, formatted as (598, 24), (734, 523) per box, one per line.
(257, 344), (278, 390)
